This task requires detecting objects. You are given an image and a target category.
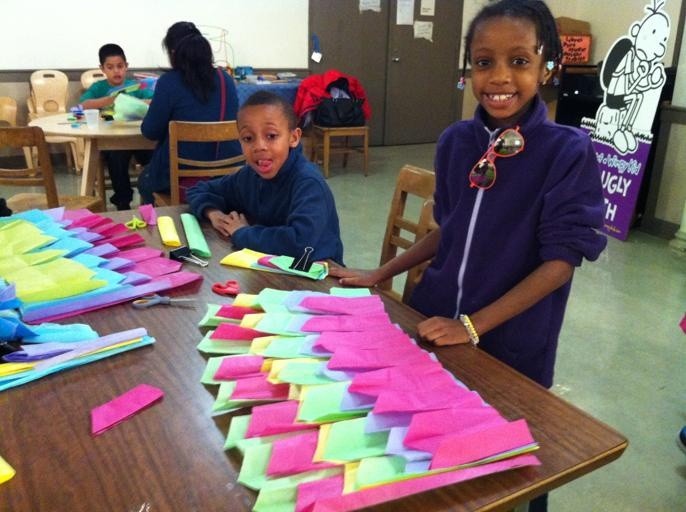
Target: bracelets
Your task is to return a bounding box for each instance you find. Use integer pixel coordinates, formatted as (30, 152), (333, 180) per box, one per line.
(459, 313), (480, 346)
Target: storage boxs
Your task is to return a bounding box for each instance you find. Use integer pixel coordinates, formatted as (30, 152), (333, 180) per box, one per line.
(554, 16), (593, 65)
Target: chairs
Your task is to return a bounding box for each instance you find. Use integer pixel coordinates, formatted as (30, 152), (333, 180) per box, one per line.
(403, 199), (441, 305)
(95, 157), (143, 207)
(26, 69), (85, 176)
(80, 69), (108, 90)
(151, 119), (248, 207)
(0, 95), (36, 177)
(377, 163), (437, 305)
(0, 126), (106, 214)
(310, 123), (369, 179)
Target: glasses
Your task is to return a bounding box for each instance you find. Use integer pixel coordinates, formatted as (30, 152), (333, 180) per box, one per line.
(469, 126), (526, 189)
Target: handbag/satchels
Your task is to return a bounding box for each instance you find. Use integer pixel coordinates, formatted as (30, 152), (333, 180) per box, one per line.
(178, 178), (211, 204)
(310, 77), (368, 126)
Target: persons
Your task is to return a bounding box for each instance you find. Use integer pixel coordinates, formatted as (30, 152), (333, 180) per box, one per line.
(327, 0), (608, 388)
(676, 315), (686, 453)
(137, 21), (244, 205)
(185, 92), (343, 264)
(79, 44), (154, 211)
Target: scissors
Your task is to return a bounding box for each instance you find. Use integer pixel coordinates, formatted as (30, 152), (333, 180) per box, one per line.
(124, 214), (147, 230)
(212, 280), (242, 295)
(132, 292), (196, 308)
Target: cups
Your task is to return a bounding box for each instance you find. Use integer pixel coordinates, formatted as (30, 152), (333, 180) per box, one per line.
(84, 110), (99, 130)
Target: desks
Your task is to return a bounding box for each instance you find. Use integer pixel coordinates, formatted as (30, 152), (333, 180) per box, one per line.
(0, 204), (628, 512)
(27, 109), (159, 197)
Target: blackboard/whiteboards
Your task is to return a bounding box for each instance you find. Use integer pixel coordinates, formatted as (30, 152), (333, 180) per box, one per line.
(0, 0), (310, 72)
